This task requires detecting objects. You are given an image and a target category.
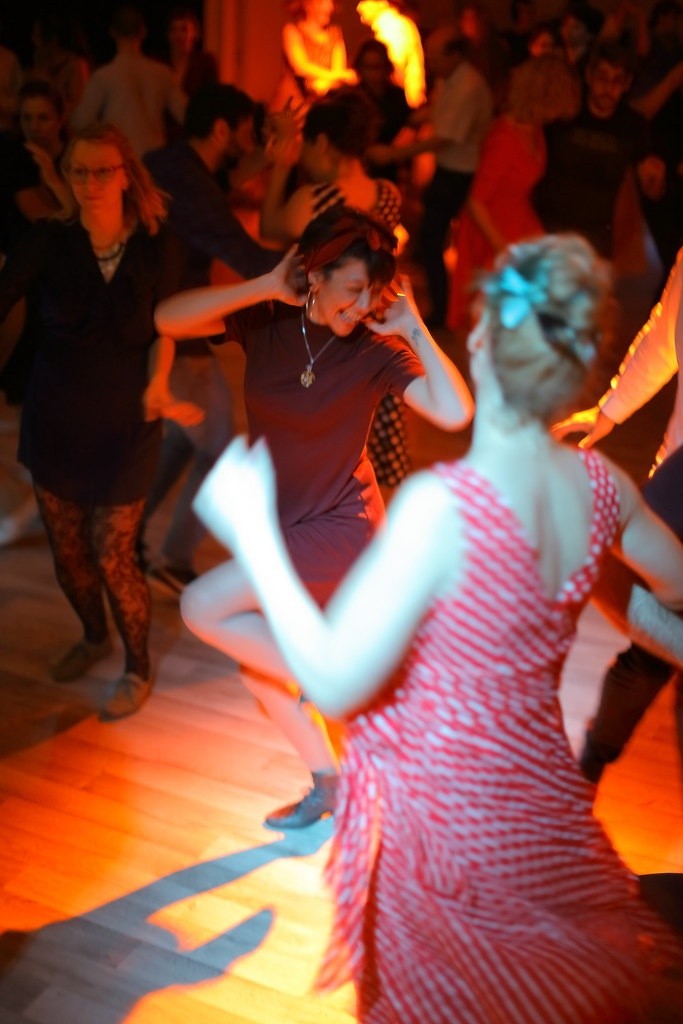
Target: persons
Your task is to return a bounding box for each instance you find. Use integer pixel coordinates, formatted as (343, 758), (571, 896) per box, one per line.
(135, 84), (402, 597)
(278, 0), (683, 336)
(0, 0), (220, 405)
(551, 242), (683, 784)
(190, 235), (683, 1024)
(0, 121), (206, 719)
(154, 206), (475, 830)
(441, 38), (665, 346)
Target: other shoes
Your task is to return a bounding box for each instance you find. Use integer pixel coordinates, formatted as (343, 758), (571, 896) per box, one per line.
(51, 642), (112, 683)
(103, 658), (158, 717)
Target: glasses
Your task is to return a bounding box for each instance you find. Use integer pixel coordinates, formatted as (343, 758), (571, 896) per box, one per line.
(64, 161), (124, 187)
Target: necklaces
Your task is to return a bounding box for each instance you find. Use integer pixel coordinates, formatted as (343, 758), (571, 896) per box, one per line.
(301, 313), (336, 388)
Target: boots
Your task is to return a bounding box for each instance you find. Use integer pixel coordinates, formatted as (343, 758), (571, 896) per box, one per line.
(267, 773), (341, 829)
